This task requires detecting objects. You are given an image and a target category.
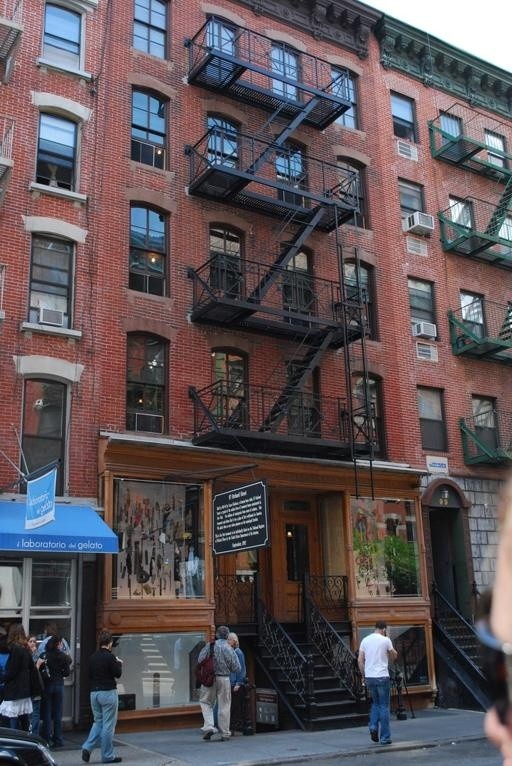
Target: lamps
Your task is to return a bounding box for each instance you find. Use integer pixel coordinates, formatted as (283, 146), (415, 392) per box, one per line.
(439, 488), (448, 498)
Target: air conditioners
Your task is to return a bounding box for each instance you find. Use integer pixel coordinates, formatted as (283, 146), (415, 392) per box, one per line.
(405, 211), (435, 235)
(37, 307), (64, 327)
(413, 319), (437, 338)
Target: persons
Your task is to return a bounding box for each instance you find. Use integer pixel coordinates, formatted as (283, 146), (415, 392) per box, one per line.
(0, 622), (72, 748)
(198, 626), (241, 741)
(81, 628), (122, 764)
(358, 621), (397, 744)
(474, 468), (512, 766)
(214, 632), (246, 737)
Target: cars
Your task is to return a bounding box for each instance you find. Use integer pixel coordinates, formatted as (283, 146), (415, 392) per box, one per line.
(0, 725), (57, 766)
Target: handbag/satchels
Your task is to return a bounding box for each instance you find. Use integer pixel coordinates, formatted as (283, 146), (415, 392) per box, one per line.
(40, 664), (50, 679)
(195, 656), (215, 687)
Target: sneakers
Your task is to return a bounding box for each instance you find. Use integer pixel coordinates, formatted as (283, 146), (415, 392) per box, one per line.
(106, 757), (121, 763)
(222, 737), (229, 741)
(371, 729), (378, 742)
(203, 730), (213, 739)
(82, 749), (90, 761)
